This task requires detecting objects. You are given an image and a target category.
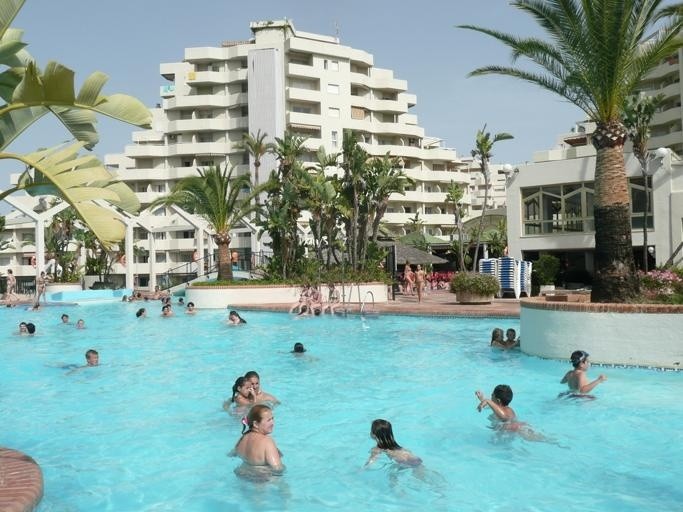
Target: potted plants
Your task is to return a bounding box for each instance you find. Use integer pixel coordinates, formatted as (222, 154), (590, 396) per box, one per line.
(531, 252), (561, 297)
(446, 269), (502, 306)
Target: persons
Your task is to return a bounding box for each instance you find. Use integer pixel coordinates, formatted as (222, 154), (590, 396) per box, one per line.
(7, 256), (84, 336)
(44, 349), (99, 375)
(557, 350), (608, 403)
(232, 377), (257, 416)
(122, 285), (196, 318)
(289, 283), (340, 321)
(223, 371), (281, 410)
(475, 384), (547, 442)
(290, 343), (307, 357)
(491, 328), (520, 349)
(506, 328), (520, 347)
(231, 248), (239, 266)
(397, 259), (426, 304)
(364, 418), (425, 487)
(232, 313), (246, 324)
(228, 310), (236, 324)
(226, 404), (291, 499)
